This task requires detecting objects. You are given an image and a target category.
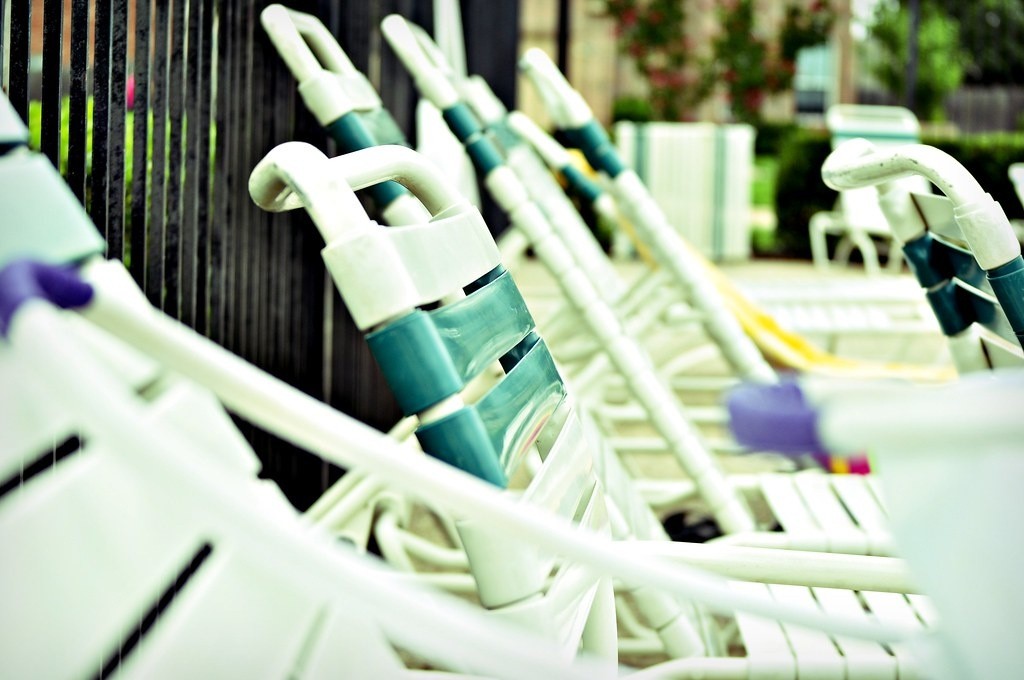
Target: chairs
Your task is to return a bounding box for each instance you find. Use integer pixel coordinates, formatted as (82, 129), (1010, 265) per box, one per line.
(0, 0), (1024, 680)
